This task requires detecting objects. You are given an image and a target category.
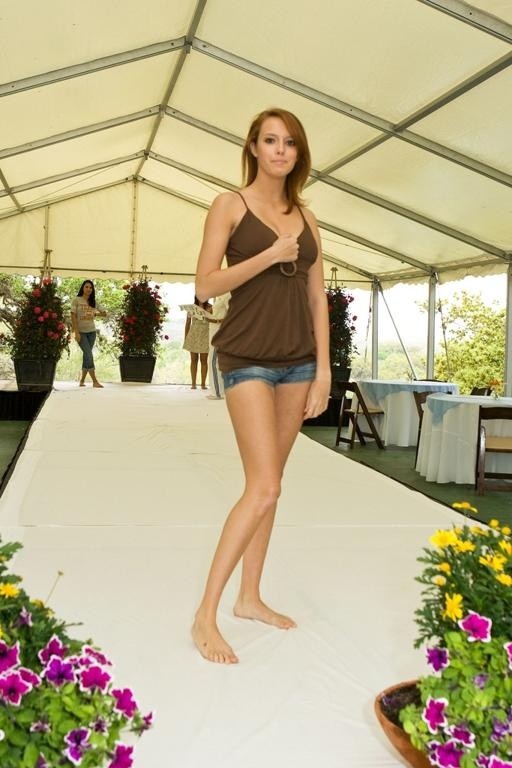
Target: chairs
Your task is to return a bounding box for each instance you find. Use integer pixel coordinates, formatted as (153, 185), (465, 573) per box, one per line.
(413, 379), (447, 383)
(334, 381), (383, 449)
(412, 389), (453, 467)
(473, 400), (512, 496)
(470, 385), (489, 396)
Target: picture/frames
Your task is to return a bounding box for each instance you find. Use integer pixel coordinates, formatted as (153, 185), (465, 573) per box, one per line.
(117, 355), (156, 382)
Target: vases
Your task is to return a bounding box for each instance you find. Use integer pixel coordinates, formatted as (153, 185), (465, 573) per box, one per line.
(490, 391), (500, 400)
(377, 680), (438, 766)
(10, 358), (57, 392)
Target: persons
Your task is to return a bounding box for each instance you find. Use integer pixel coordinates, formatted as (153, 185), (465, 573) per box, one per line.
(69, 280), (108, 388)
(183, 293), (213, 389)
(186, 110), (332, 665)
(200, 292), (231, 401)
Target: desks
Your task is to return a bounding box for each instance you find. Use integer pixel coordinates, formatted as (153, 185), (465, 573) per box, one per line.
(349, 382), (458, 448)
(415, 395), (512, 489)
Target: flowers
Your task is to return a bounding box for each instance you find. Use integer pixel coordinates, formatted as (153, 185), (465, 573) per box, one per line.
(322, 276), (358, 397)
(5, 272), (72, 357)
(112, 277), (171, 355)
(0, 537), (153, 766)
(488, 375), (504, 392)
(406, 500), (512, 767)
